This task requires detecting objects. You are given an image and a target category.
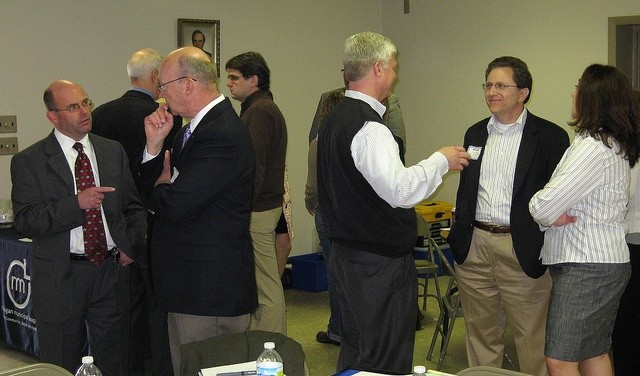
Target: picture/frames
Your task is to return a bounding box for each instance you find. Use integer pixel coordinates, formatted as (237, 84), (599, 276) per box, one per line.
(177, 18), (220, 80)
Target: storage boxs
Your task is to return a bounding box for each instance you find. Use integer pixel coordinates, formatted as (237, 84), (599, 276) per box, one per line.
(289, 252), (328, 293)
(412, 244), (453, 279)
(414, 200), (453, 247)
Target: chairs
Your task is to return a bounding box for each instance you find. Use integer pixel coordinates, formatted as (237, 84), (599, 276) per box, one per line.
(427, 237), (518, 372)
(416, 213), (444, 314)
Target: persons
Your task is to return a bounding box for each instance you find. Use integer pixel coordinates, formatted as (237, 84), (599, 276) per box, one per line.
(305, 137), (348, 349)
(275, 211), (292, 278)
(89, 48), (183, 192)
(528, 64), (634, 375)
(191, 30), (212, 60)
(9, 82), (150, 376)
(319, 32), (470, 376)
(227, 52), (287, 343)
(447, 57), (571, 376)
(135, 48), (259, 375)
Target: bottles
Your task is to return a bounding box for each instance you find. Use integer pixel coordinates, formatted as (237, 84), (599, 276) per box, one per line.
(256, 341), (284, 375)
(413, 364), (428, 375)
(76, 357), (101, 376)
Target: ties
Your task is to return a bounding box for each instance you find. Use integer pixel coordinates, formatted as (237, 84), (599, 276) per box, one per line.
(73, 142), (109, 268)
(181, 125), (191, 146)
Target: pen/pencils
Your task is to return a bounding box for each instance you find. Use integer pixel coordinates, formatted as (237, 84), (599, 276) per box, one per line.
(216, 370), (257, 376)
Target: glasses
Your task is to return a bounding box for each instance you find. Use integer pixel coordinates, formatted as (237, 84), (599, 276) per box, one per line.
(481, 82), (521, 90)
(227, 75), (244, 81)
(156, 76), (198, 91)
(49, 98), (92, 111)
